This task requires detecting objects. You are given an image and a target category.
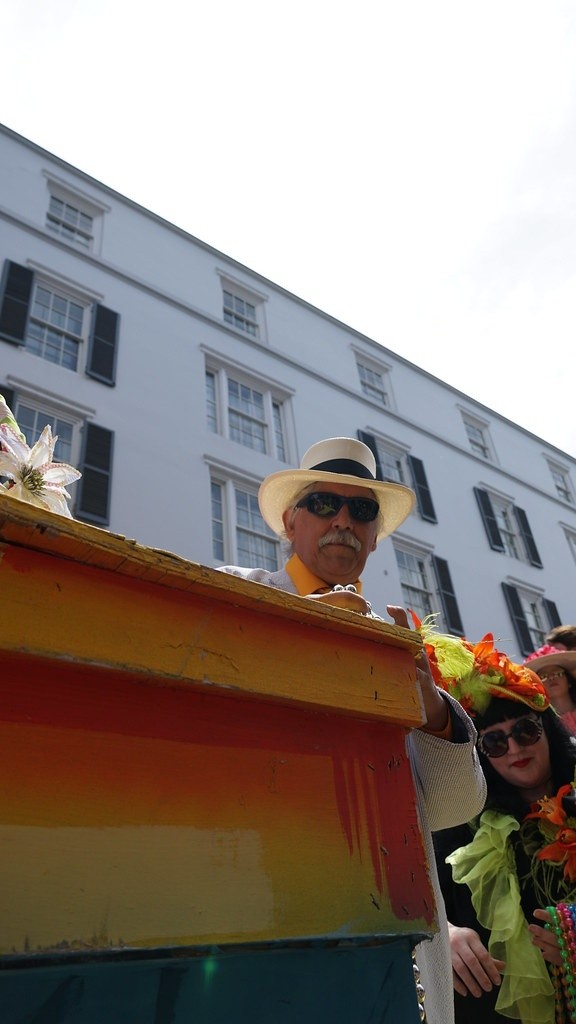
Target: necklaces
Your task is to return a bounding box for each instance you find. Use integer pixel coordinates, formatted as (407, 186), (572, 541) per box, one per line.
(545, 902), (576, 1024)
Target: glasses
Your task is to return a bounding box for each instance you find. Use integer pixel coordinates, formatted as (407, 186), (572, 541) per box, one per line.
(477, 713), (544, 758)
(539, 671), (568, 682)
(293, 491), (380, 523)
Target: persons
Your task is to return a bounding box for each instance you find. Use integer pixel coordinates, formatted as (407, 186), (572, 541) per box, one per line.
(430, 633), (576, 1024)
(519, 626), (576, 740)
(212, 436), (488, 1024)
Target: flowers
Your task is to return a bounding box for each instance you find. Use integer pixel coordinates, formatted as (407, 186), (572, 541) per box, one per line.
(523, 785), (576, 885)
(406, 608), (548, 721)
(0, 393), (81, 520)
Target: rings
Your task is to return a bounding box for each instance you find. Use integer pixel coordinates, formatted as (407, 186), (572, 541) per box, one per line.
(414, 648), (424, 660)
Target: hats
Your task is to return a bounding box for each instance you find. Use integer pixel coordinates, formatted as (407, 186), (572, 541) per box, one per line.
(522, 644), (576, 675)
(407, 607), (550, 711)
(258, 439), (416, 542)
